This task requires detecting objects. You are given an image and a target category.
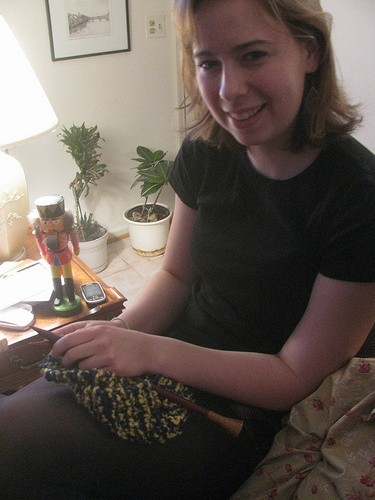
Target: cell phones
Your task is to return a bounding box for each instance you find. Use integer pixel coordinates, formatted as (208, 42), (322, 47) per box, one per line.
(79, 281), (107, 305)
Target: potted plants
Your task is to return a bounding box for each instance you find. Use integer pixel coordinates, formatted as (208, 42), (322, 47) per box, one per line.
(122, 145), (174, 257)
(56, 121), (109, 273)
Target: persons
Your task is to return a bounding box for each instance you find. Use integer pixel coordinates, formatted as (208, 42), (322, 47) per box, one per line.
(0, 0), (375, 500)
(29, 195), (81, 305)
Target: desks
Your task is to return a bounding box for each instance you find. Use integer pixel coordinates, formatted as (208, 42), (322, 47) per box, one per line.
(0, 225), (127, 396)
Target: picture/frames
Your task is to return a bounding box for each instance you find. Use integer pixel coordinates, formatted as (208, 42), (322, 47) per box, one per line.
(45, 0), (131, 61)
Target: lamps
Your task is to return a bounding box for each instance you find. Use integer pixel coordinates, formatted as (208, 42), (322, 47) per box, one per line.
(0, 16), (59, 263)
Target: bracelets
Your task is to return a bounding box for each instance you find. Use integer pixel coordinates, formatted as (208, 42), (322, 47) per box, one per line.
(109, 316), (131, 332)
